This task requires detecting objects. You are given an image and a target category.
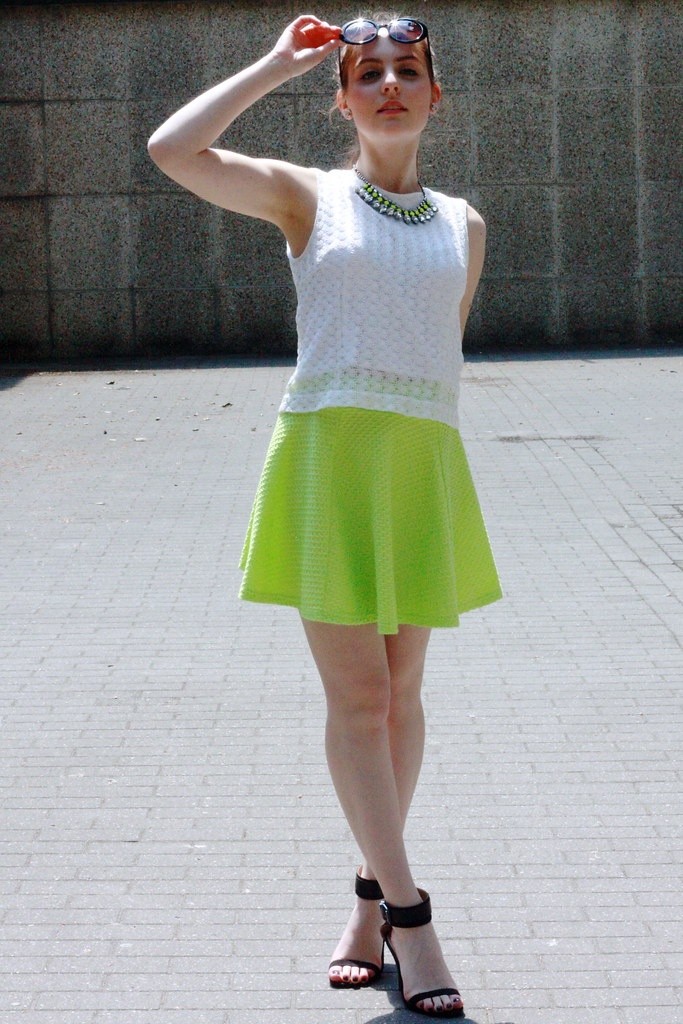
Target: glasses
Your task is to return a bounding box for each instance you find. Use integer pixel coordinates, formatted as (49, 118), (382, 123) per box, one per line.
(338, 18), (434, 87)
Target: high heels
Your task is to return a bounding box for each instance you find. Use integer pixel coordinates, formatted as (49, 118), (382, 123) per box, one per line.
(380, 886), (465, 1019)
(328, 864), (386, 988)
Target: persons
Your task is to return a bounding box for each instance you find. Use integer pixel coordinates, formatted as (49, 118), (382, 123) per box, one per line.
(145, 15), (505, 1019)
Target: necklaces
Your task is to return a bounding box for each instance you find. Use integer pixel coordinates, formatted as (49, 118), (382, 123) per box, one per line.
(350, 164), (440, 225)
